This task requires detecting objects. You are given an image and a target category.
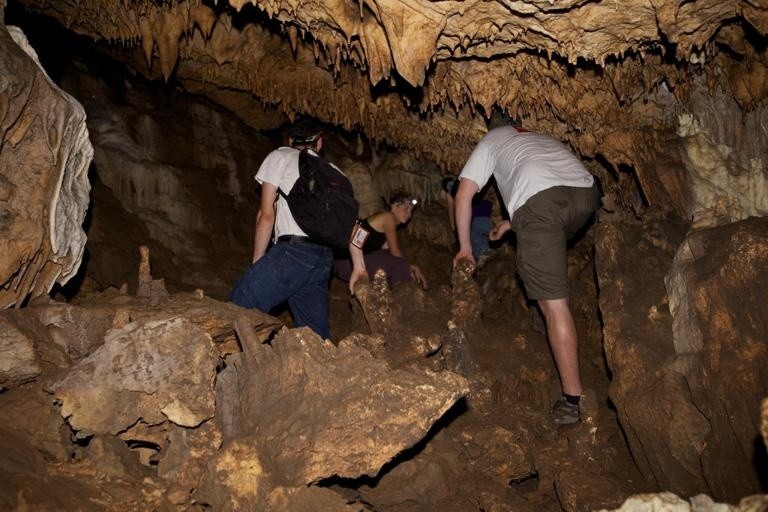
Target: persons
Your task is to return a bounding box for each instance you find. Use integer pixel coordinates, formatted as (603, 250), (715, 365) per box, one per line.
(452, 106), (601, 426)
(228, 117), (370, 343)
(334, 192), (428, 290)
(442, 177), (508, 257)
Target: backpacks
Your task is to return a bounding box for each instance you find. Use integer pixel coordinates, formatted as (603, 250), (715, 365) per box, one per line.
(278, 147), (359, 250)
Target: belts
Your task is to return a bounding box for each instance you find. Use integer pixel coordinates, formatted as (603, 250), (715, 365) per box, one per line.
(278, 235), (313, 243)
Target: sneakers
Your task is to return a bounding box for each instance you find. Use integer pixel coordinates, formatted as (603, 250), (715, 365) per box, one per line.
(550, 397), (580, 425)
